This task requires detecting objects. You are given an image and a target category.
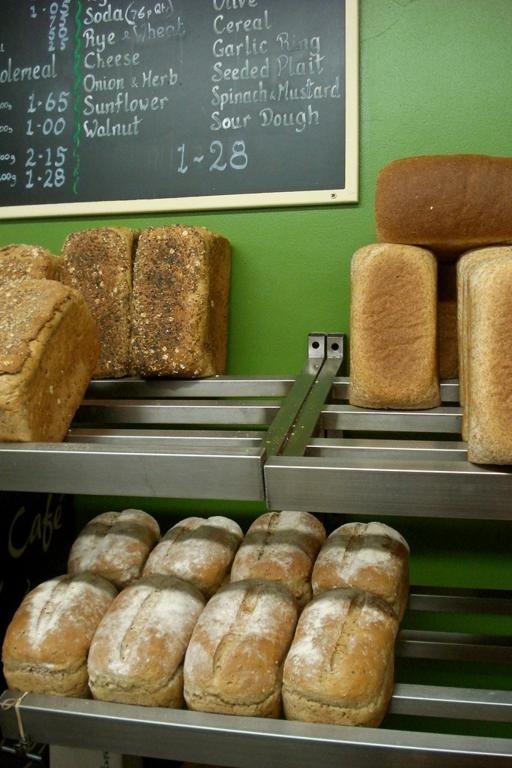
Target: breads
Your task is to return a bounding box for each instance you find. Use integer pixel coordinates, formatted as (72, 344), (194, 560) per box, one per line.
(434, 252), (460, 384)
(86, 575), (208, 710)
(1, 278), (102, 443)
(346, 242), (442, 409)
(0, 242), (70, 285)
(59, 225), (142, 378)
(182, 578), (302, 719)
(375, 154), (512, 247)
(138, 515), (244, 606)
(229, 508), (324, 613)
(456, 244), (512, 465)
(67, 506), (160, 593)
(281, 585), (399, 727)
(309, 520), (411, 627)
(133, 224), (231, 379)
(1, 572), (120, 699)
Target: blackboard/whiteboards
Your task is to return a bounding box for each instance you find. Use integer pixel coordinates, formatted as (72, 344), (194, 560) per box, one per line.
(1, 0), (358, 217)
